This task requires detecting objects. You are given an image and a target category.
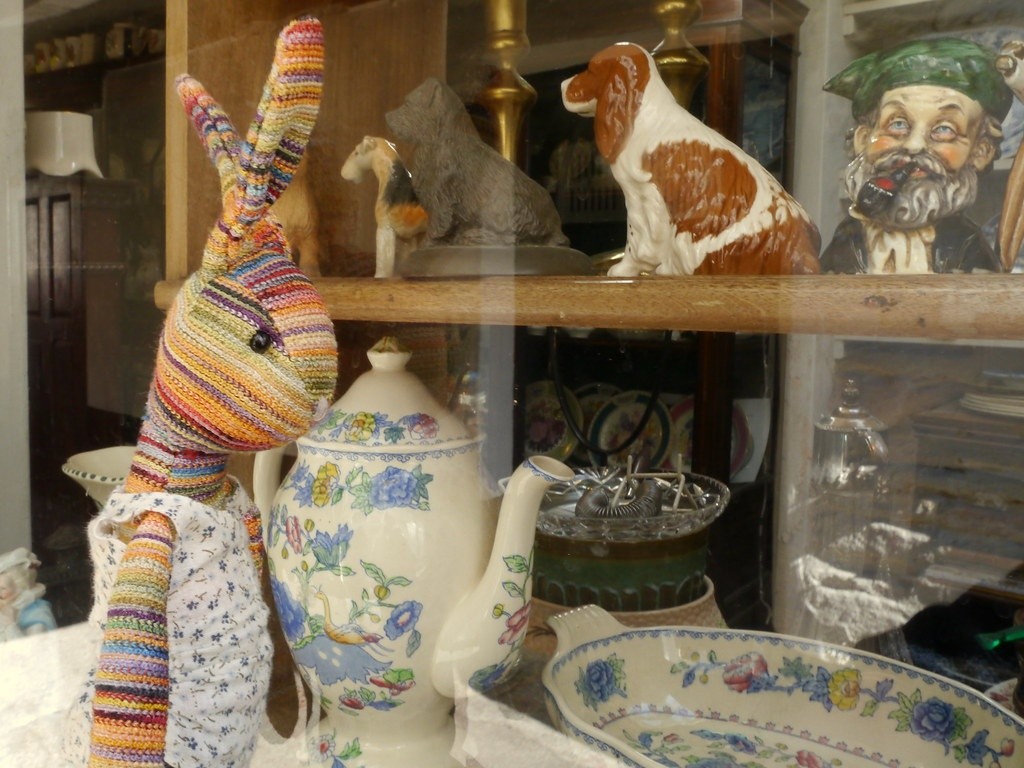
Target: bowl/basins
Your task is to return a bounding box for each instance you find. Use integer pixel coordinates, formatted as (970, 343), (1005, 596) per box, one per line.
(65, 447), (140, 505)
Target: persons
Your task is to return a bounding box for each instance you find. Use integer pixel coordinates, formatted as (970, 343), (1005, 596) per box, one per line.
(820, 36), (1013, 276)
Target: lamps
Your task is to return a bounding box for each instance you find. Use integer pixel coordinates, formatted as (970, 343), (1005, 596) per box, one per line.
(24, 111), (106, 177)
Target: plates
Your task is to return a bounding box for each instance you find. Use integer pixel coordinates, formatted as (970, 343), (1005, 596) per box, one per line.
(983, 676), (1018, 715)
(537, 605), (1024, 768)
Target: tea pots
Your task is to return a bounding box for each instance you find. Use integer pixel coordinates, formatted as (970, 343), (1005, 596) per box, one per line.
(254, 337), (574, 768)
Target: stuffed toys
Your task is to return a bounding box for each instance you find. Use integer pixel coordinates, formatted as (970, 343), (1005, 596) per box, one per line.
(90, 16), (338, 768)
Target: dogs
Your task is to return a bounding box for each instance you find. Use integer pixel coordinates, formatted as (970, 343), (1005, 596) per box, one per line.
(384, 76), (571, 246)
(560, 42), (821, 275)
(341, 135), (429, 279)
